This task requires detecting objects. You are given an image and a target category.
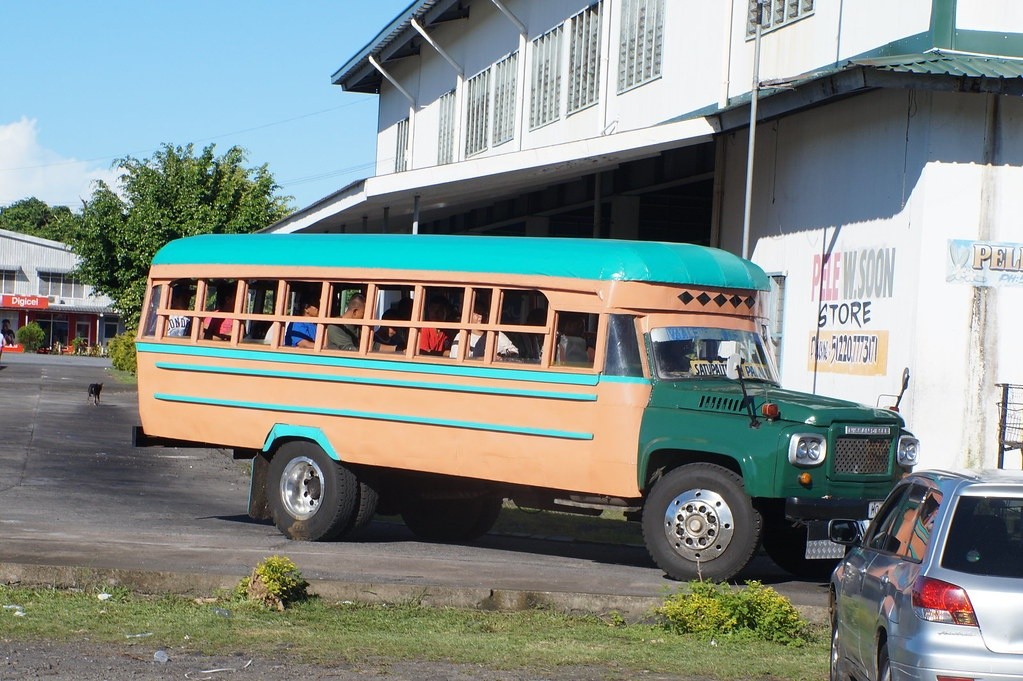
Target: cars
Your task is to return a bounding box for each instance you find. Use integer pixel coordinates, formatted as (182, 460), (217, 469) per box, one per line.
(825, 467), (1023, 681)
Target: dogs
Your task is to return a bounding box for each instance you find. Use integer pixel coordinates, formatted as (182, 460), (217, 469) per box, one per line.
(88, 382), (103, 406)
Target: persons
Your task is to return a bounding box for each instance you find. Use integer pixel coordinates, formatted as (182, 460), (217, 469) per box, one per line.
(285, 297), (320, 348)
(450, 307), (519, 361)
(514, 308), (546, 362)
(0, 331), (6, 361)
(326, 292), (366, 351)
(419, 303), (451, 356)
(554, 316), (593, 368)
(373, 307), (407, 354)
(150, 284), (192, 336)
(1, 318), (15, 346)
(202, 292), (246, 341)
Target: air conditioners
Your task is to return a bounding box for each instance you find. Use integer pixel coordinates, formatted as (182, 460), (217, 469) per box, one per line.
(47, 294), (61, 305)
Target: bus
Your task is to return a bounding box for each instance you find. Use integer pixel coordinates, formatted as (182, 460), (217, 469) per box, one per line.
(129, 229), (922, 588)
